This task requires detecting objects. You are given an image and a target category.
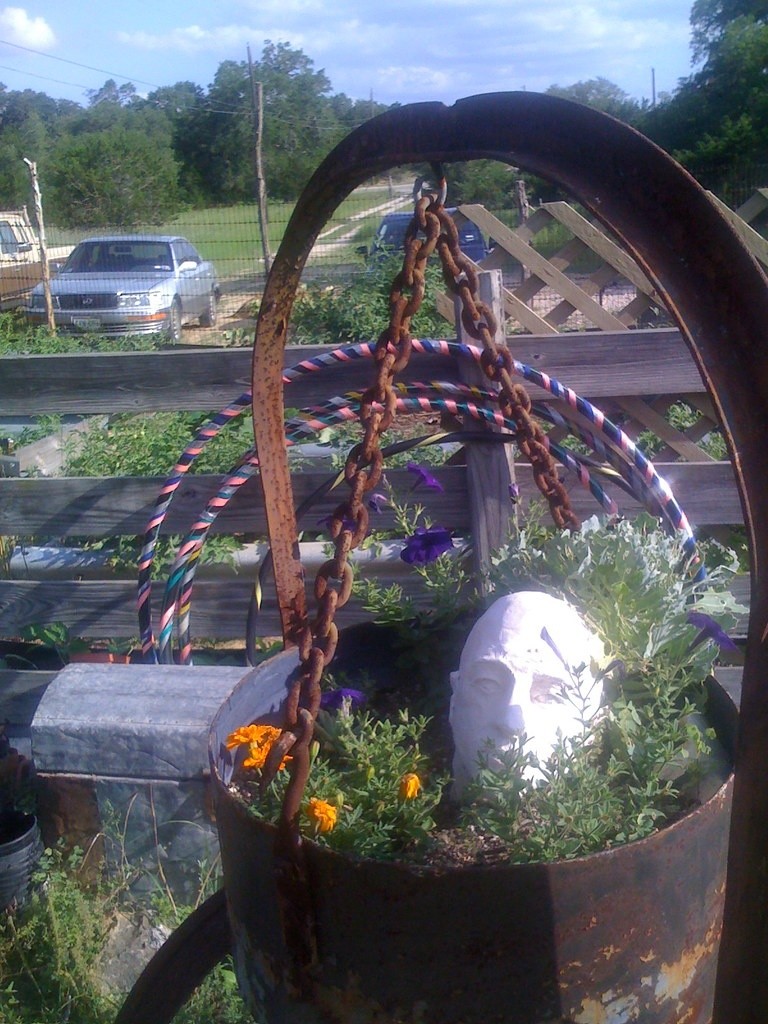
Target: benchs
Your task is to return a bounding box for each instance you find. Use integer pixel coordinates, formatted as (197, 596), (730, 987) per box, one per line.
(0, 328), (768, 906)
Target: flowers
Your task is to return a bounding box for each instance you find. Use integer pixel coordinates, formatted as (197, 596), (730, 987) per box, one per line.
(213, 425), (751, 869)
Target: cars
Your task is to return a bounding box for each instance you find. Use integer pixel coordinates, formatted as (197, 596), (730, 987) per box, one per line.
(22, 235), (222, 344)
(353, 207), (488, 276)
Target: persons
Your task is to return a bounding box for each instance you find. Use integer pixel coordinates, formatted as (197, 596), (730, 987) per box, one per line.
(449, 591), (602, 793)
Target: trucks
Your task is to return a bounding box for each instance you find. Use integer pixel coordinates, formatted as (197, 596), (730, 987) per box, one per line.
(0, 205), (76, 268)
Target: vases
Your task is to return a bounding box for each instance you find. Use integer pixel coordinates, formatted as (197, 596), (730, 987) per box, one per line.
(204, 611), (742, 1024)
